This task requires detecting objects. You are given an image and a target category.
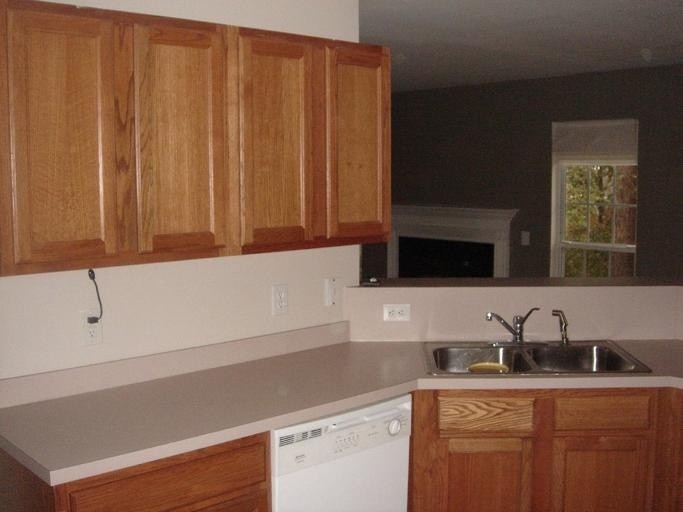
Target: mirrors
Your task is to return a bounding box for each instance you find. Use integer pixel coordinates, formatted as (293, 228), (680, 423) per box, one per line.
(357, 0), (683, 288)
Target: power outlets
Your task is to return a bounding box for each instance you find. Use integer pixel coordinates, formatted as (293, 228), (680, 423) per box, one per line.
(79, 309), (103, 346)
(271, 283), (289, 316)
(383, 304), (411, 322)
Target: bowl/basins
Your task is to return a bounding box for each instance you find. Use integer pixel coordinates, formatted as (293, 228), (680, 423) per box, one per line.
(468, 362), (509, 374)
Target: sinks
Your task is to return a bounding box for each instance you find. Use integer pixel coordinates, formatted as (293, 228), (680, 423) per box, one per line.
(520, 338), (653, 378)
(421, 341), (533, 377)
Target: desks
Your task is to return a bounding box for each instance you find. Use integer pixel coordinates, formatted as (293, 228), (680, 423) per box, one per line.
(385, 204), (520, 279)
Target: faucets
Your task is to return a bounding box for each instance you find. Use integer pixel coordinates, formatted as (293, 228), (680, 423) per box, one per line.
(485, 307), (540, 344)
(551, 310), (569, 343)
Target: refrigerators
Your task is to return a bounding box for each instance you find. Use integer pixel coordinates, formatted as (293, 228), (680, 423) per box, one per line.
(272, 392), (411, 512)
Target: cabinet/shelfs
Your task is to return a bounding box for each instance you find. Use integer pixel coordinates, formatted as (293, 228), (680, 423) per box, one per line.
(0, 432), (272, 512)
(407, 387), (683, 512)
(230, 25), (392, 255)
(0, 0), (230, 277)
